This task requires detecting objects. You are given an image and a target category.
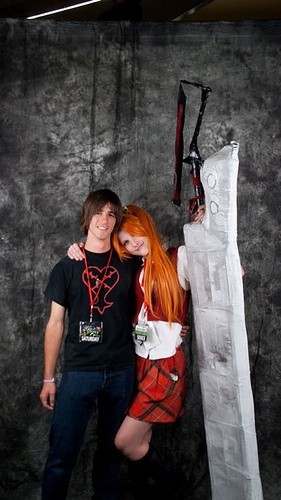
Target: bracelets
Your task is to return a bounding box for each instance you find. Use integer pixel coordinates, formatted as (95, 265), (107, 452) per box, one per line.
(44, 378), (55, 383)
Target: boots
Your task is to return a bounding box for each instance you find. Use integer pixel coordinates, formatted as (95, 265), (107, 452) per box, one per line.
(131, 442), (190, 498)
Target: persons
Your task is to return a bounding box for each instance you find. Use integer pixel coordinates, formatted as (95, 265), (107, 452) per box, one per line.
(67, 203), (208, 500)
(41, 190), (190, 500)
